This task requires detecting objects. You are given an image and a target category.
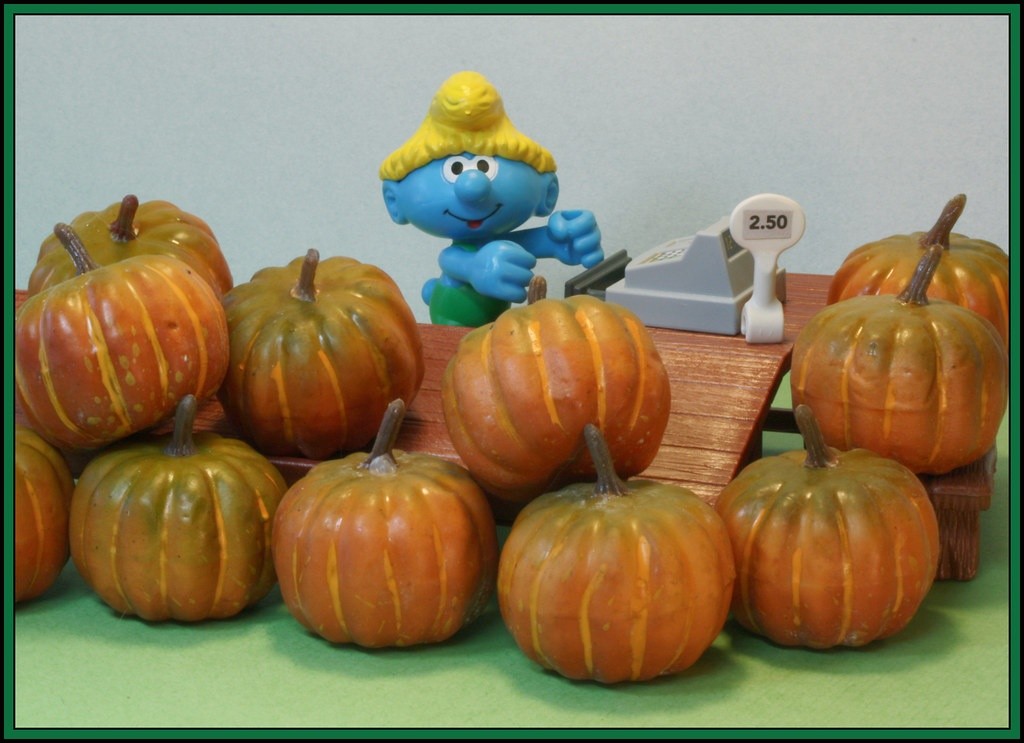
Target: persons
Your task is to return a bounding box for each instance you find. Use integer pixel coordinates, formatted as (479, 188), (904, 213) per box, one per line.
(380, 68), (603, 327)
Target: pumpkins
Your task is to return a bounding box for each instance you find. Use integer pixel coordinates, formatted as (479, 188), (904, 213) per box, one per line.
(15, 192), (1006, 682)
